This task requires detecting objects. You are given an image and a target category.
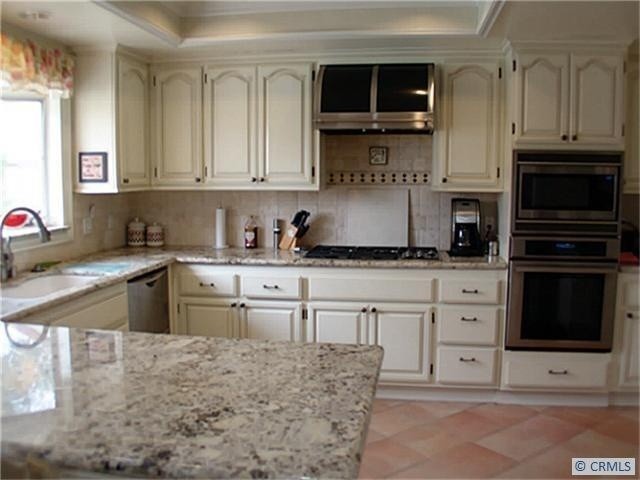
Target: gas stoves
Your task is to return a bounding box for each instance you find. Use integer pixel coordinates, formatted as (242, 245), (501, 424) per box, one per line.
(303, 245), (440, 260)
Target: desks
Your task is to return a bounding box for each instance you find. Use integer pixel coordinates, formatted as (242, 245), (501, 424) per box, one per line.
(1, 321), (385, 480)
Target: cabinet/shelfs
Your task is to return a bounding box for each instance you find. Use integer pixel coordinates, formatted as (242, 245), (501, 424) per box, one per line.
(433, 62), (503, 193)
(617, 280), (640, 387)
(308, 273), (435, 383)
(511, 53), (624, 145)
(118, 58), (149, 186)
(205, 64), (311, 183)
(149, 68), (201, 185)
(173, 273), (303, 341)
(502, 349), (612, 391)
(47, 292), (129, 333)
(437, 275), (502, 385)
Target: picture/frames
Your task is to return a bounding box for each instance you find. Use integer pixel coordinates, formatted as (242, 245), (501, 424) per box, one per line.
(79, 151), (107, 183)
(369, 145), (390, 165)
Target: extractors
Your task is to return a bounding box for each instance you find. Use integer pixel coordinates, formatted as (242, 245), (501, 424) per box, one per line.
(312, 64), (435, 134)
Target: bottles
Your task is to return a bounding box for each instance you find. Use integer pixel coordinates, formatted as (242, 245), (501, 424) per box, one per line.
(244, 215), (258, 249)
(489, 235), (499, 256)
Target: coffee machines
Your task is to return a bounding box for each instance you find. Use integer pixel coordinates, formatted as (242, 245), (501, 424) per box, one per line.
(450, 197), (486, 258)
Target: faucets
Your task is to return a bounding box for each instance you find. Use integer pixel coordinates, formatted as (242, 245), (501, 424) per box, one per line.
(0, 206), (51, 283)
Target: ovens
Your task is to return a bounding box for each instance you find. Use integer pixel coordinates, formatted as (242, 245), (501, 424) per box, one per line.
(505, 145), (625, 352)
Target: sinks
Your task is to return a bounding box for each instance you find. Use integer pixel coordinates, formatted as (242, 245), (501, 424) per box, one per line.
(0, 272), (103, 300)
